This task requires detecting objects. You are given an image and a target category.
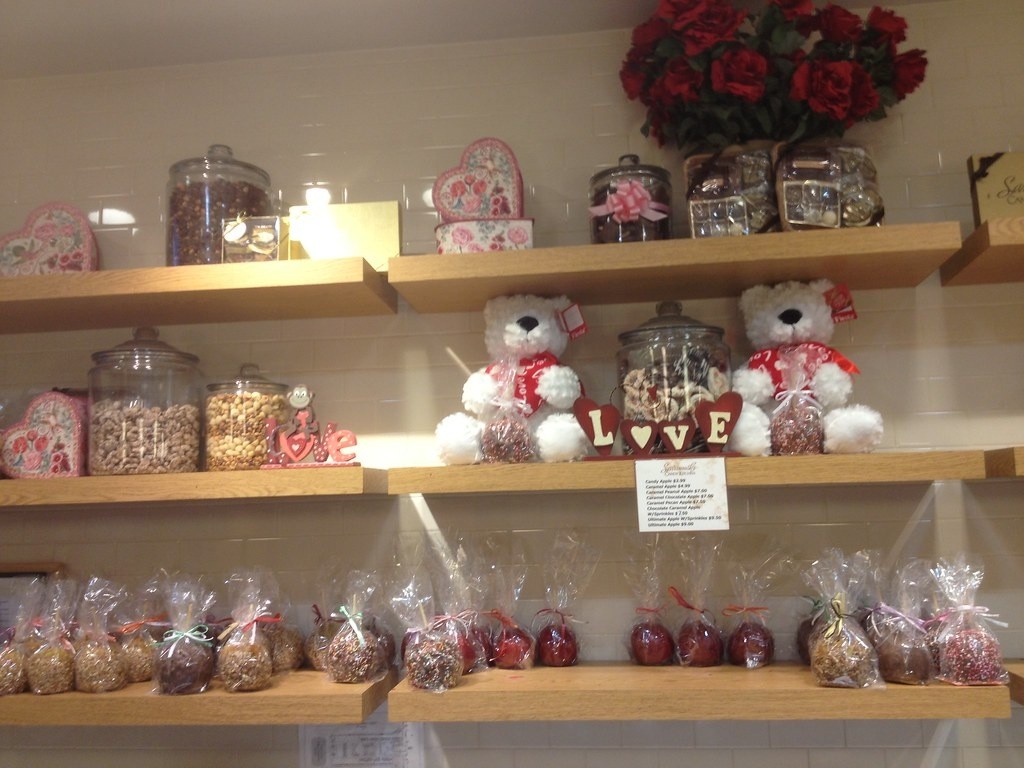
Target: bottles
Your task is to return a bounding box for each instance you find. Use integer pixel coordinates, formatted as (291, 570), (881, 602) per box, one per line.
(87, 328), (202, 475)
(588, 154), (673, 243)
(165, 144), (273, 269)
(617, 296), (731, 458)
(203, 363), (294, 471)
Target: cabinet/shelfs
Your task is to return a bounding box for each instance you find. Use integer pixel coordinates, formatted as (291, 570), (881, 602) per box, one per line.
(0, 219), (1024, 720)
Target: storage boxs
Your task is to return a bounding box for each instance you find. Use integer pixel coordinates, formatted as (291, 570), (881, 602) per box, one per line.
(287, 201), (403, 276)
(966, 151), (1024, 229)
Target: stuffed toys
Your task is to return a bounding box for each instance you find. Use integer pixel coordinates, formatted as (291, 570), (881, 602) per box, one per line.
(724, 278), (887, 458)
(434, 295), (593, 466)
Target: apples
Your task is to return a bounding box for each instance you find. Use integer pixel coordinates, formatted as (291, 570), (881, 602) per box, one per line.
(631, 617), (820, 666)
(402, 623), (577, 673)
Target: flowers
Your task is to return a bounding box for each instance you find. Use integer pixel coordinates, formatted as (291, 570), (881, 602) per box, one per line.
(619, 0), (929, 161)
